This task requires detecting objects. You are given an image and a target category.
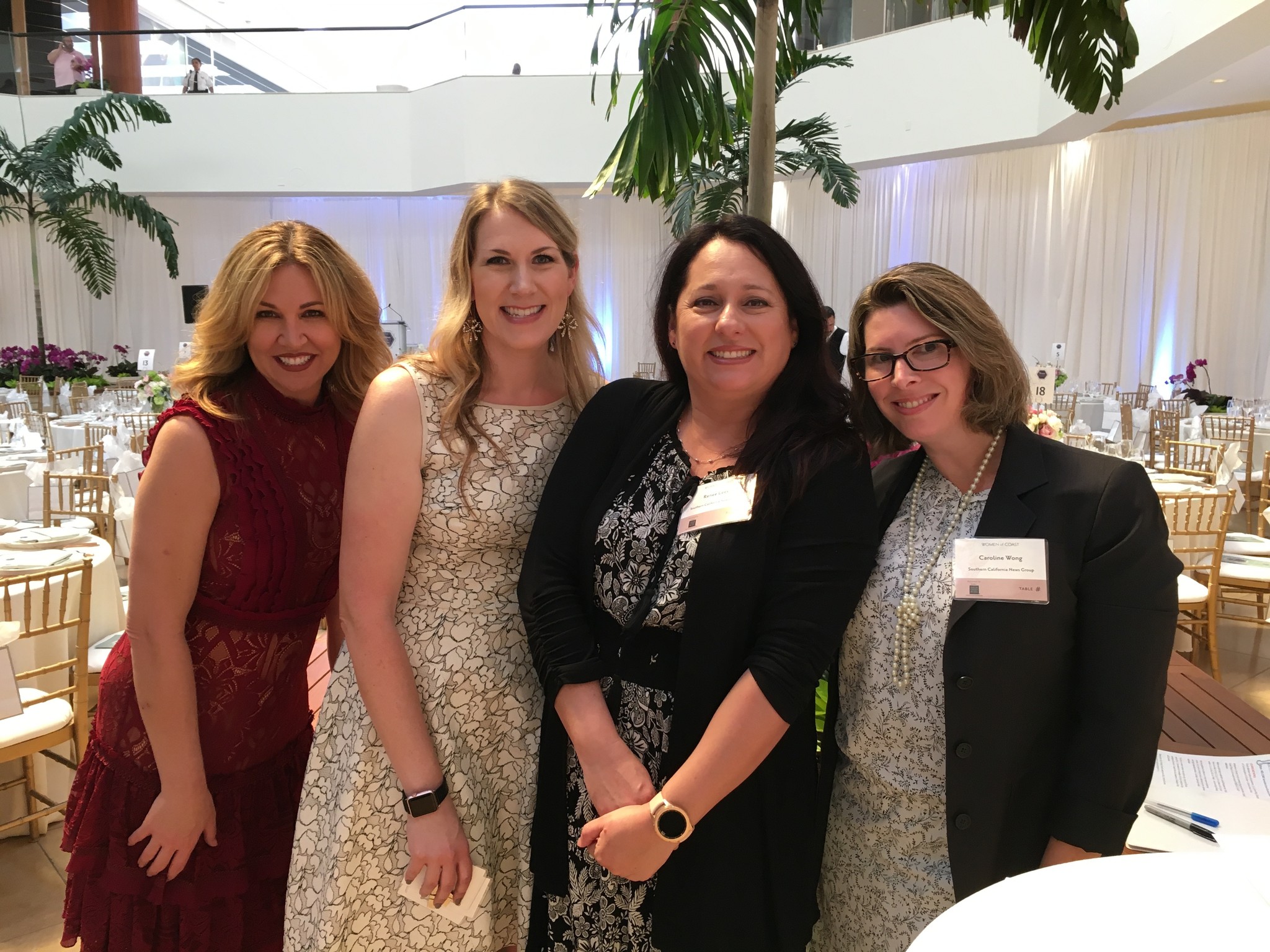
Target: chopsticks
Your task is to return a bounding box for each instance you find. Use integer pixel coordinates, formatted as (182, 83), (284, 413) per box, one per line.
(43, 542), (100, 548)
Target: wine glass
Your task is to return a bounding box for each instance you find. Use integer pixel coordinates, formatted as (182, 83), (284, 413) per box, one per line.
(1068, 378), (1103, 401)
(1227, 400), (1236, 424)
(1231, 396), (1242, 417)
(1242, 398), (1254, 418)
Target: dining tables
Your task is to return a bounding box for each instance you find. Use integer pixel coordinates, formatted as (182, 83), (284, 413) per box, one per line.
(0, 443), (65, 525)
(1176, 414), (1270, 473)
(0, 393), (33, 419)
(1147, 473), (1220, 653)
(1038, 396), (1105, 432)
(49, 412), (158, 460)
(0, 523), (127, 843)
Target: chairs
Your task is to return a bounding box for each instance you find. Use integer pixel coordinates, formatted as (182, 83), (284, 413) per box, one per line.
(0, 372), (221, 844)
(1021, 382), (1270, 685)
(633, 362), (670, 382)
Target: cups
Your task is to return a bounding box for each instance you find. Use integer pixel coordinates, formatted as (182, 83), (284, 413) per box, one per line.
(1131, 450), (1146, 468)
(1118, 439), (1133, 461)
(1089, 435), (1106, 454)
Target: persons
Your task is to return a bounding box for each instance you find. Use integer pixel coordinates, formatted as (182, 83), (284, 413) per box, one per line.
(807, 262), (1185, 952)
(518, 215), (871, 952)
(182, 58), (215, 94)
(824, 304), (851, 387)
(47, 35), (89, 96)
(285, 180), (606, 951)
(59, 222), (392, 952)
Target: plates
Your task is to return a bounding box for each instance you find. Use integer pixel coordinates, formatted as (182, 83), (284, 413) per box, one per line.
(59, 416), (84, 419)
(0, 529), (90, 548)
(56, 421), (82, 425)
(0, 553), (84, 575)
(85, 422), (104, 425)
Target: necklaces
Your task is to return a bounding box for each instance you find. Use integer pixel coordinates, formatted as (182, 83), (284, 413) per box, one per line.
(677, 418), (741, 463)
(891, 424), (1004, 687)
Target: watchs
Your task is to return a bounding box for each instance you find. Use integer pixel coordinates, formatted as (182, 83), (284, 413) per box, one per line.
(402, 777), (449, 818)
(649, 791), (695, 843)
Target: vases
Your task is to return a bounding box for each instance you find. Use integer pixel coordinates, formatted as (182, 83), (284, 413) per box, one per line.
(75, 88), (116, 97)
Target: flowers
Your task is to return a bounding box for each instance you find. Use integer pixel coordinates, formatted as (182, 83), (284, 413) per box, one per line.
(1163, 357), (1214, 396)
(1032, 357), (1070, 388)
(135, 371), (171, 415)
(0, 345), (113, 391)
(70, 54), (111, 94)
(1024, 404), (1065, 443)
(106, 343), (141, 377)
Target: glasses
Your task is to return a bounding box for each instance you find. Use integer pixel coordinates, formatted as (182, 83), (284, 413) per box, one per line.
(849, 339), (958, 382)
